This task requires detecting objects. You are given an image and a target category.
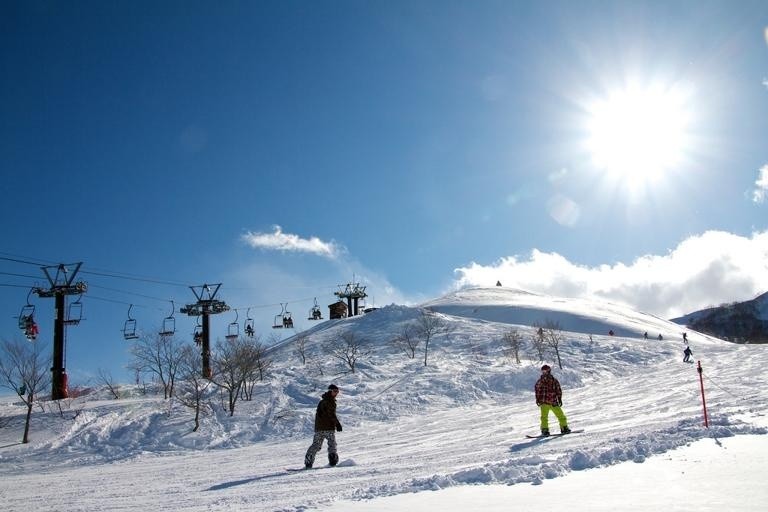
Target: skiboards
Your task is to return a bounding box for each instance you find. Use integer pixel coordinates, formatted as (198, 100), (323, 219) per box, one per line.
(683, 360), (695, 363)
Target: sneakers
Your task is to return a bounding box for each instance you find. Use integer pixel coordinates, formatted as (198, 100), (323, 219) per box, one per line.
(561, 425), (571, 434)
(541, 428), (550, 436)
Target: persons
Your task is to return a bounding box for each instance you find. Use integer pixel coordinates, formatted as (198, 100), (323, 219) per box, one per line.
(534, 365), (571, 435)
(24, 320), (39, 339)
(247, 324), (254, 337)
(316, 308), (323, 319)
(644, 332), (648, 339)
(657, 333), (663, 340)
(683, 346), (692, 362)
(303, 383), (342, 469)
(313, 309), (317, 320)
(194, 330), (201, 347)
(536, 328), (545, 342)
(283, 316), (288, 328)
(682, 331), (688, 343)
(608, 330), (614, 337)
(287, 317), (292, 327)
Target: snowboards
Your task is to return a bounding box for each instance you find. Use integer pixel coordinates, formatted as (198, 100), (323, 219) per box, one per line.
(526, 430), (583, 439)
(287, 458), (352, 471)
